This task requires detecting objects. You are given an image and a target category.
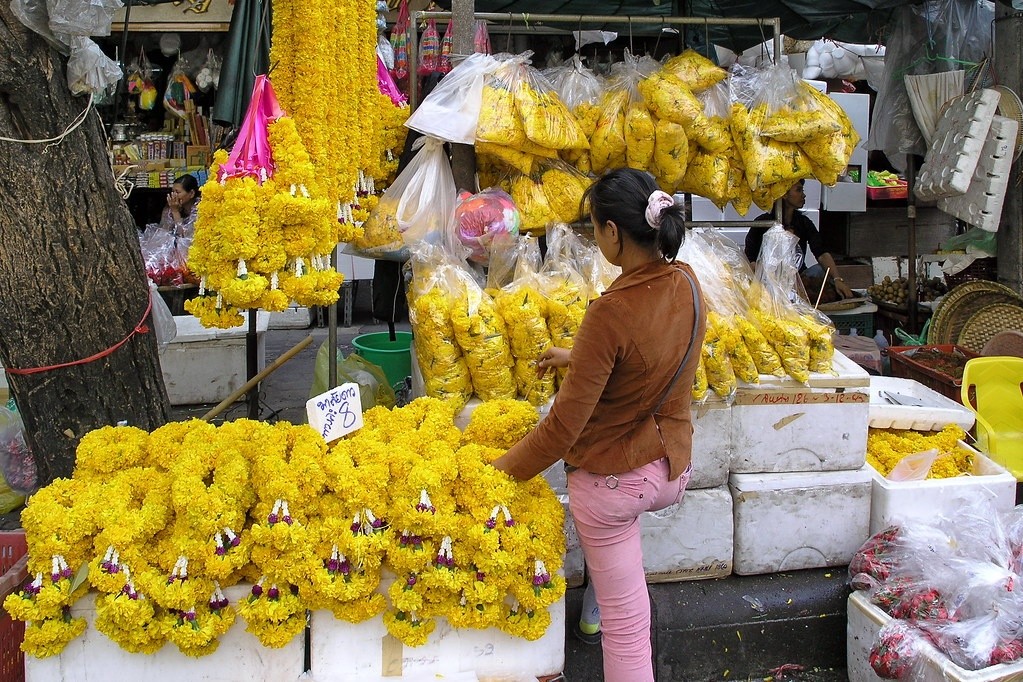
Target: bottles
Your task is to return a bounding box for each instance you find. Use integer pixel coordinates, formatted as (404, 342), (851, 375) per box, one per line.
(874, 330), (891, 375)
(849, 327), (858, 337)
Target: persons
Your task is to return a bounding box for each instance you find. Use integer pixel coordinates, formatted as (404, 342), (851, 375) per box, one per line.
(167, 174), (200, 245)
(744, 179), (854, 303)
(491, 166), (708, 682)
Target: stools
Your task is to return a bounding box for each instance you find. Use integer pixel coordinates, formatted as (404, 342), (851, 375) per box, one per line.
(832, 334), (882, 376)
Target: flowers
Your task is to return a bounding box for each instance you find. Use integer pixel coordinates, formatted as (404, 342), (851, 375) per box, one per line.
(866, 426), (979, 483)
(470, 49), (857, 236)
(848, 527), (1022, 680)
(184, 0), (438, 328)
(868, 169), (899, 188)
(406, 228), (836, 408)
(3, 395), (568, 655)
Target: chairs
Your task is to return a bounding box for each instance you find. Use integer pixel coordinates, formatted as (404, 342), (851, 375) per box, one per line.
(960, 357), (1023, 484)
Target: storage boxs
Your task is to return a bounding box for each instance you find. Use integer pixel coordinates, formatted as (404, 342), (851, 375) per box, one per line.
(869, 178), (909, 199)
(409, 339), (1019, 587)
(155, 298), (313, 408)
(885, 343), (988, 444)
(915, 90), (1018, 233)
(844, 584), (1023, 682)
(835, 312), (873, 337)
(1, 527), (565, 682)
(114, 145), (208, 189)
(673, 79), (872, 265)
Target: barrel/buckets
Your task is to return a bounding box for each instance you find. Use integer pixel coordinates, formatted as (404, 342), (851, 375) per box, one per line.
(351, 331), (414, 397)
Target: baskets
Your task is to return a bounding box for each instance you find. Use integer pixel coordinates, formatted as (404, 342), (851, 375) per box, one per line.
(867, 179), (908, 200)
(927, 279), (1023, 357)
(987, 85), (1023, 164)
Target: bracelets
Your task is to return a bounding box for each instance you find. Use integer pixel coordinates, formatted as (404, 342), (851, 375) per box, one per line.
(833, 277), (843, 282)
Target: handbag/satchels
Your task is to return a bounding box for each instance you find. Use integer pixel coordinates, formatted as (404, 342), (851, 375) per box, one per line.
(453, 185), (520, 267)
(309, 335), (396, 412)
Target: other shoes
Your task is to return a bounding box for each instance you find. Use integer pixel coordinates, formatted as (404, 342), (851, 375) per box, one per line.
(567, 599), (601, 645)
